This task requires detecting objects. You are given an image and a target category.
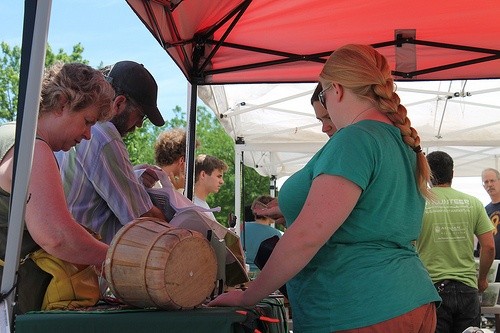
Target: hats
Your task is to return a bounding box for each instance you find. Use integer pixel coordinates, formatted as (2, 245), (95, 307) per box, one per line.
(106, 60), (166, 126)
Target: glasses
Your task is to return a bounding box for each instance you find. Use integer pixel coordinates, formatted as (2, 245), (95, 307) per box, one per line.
(317, 82), (332, 111)
(125, 97), (148, 122)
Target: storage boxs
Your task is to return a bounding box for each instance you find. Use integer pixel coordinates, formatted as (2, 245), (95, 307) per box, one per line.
(471, 258), (500, 307)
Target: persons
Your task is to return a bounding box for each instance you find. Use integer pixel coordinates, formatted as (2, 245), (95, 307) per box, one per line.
(0, 58), (111, 315)
(225, 194), (293, 319)
(411, 151), (496, 333)
(206, 43), (443, 333)
(55, 60), (167, 244)
(475, 168), (500, 333)
(153, 129), (229, 224)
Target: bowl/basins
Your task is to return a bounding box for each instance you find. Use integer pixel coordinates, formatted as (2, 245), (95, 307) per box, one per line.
(476, 282), (500, 306)
(475, 259), (500, 282)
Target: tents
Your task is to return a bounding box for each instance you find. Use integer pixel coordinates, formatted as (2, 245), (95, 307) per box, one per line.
(0, 0), (500, 333)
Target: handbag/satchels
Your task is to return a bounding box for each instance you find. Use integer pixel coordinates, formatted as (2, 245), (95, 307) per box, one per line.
(30, 248), (101, 312)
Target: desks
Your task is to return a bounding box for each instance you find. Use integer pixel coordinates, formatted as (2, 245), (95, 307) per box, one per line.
(14, 296), (288, 333)
(481, 304), (500, 333)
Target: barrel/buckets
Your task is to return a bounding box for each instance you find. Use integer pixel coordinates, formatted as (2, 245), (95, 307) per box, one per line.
(104, 217), (218, 311)
